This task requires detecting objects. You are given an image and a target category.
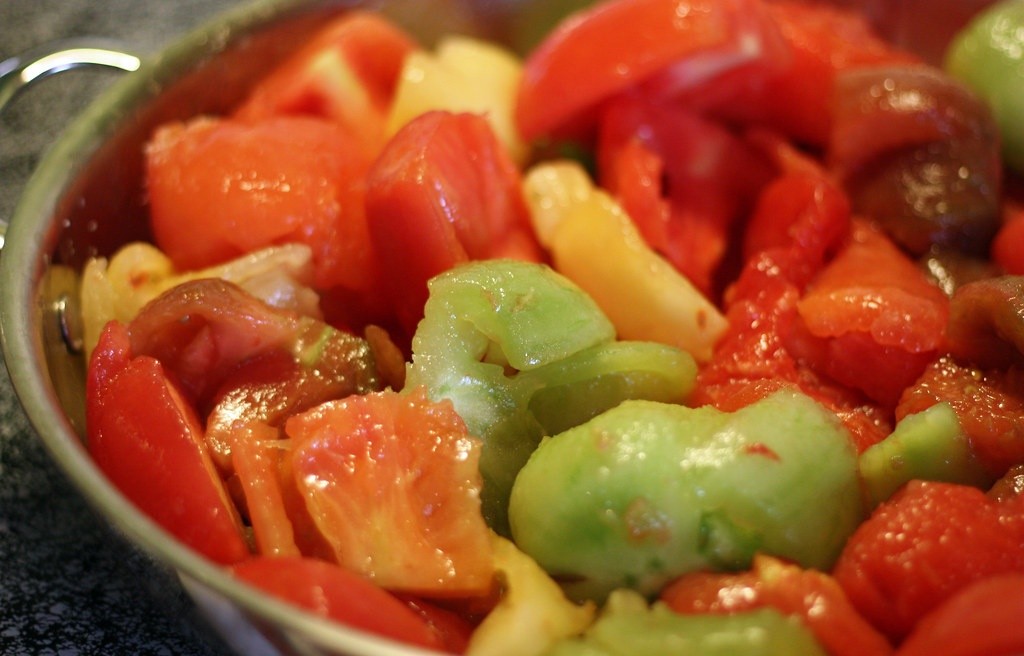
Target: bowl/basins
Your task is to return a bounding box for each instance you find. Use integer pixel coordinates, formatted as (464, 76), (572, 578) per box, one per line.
(0, 0), (594, 655)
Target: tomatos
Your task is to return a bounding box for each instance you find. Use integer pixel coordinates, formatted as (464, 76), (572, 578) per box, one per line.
(83, 3), (1024, 655)
